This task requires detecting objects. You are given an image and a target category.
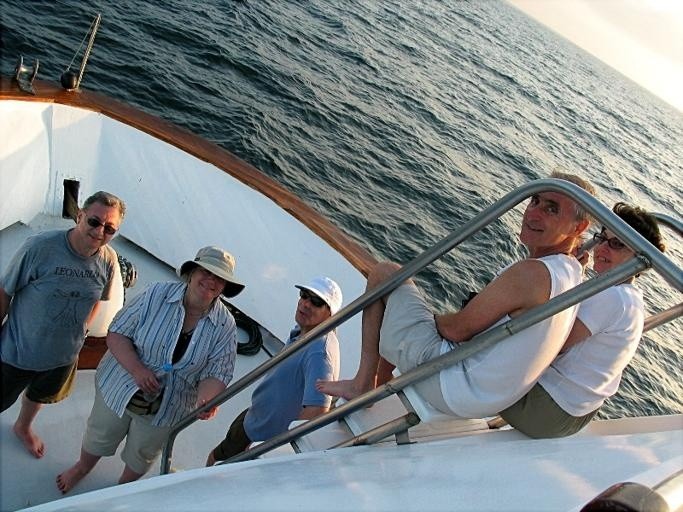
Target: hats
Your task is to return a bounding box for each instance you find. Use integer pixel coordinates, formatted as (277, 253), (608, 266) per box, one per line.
(294, 275), (343, 318)
(176, 246), (245, 298)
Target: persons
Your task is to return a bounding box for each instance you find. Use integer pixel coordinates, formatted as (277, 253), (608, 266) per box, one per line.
(498, 202), (665, 439)
(56, 246), (245, 494)
(0, 192), (125, 458)
(206, 277), (342, 467)
(315, 169), (597, 419)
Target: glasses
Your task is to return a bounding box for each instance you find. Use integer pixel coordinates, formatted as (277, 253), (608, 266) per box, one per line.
(300, 290), (324, 308)
(84, 210), (119, 235)
(593, 233), (624, 249)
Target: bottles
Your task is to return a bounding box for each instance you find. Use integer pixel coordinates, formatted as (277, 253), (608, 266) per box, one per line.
(143, 362), (174, 402)
